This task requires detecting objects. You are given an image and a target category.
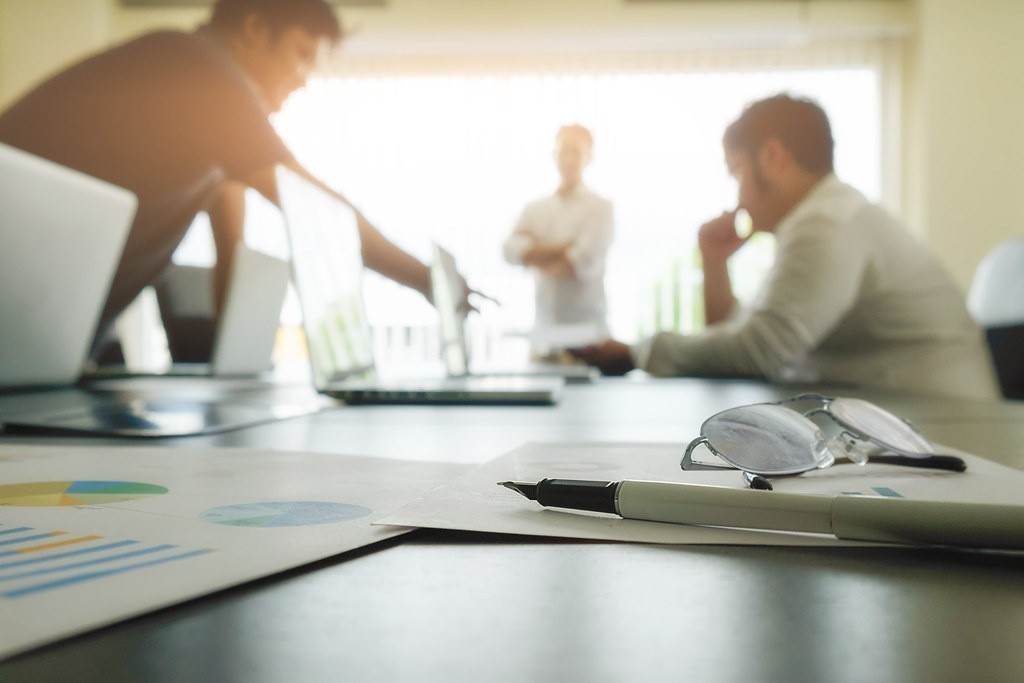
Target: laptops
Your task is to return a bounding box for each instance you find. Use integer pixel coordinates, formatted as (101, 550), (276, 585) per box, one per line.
(0, 142), (601, 404)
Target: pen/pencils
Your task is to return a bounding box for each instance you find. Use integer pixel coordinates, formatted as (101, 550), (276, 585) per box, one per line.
(495, 480), (1024, 553)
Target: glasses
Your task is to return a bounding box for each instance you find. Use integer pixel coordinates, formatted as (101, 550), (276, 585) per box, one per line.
(681, 393), (967, 492)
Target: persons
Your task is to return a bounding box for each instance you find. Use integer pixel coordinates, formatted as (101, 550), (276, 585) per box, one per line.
(567, 93), (1007, 406)
(503, 125), (617, 368)
(0, 0), (478, 379)
